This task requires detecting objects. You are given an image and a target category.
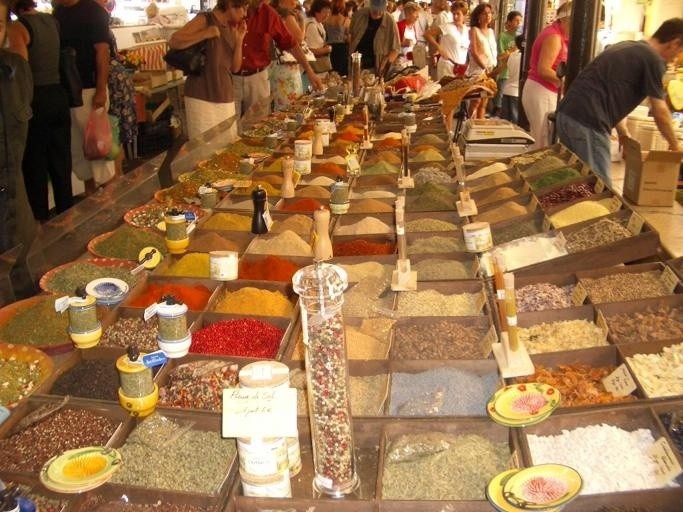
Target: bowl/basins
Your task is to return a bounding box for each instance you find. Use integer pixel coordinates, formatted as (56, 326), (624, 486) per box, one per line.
(120, 87), (309, 230)
(86, 228), (167, 264)
(0, 294), (74, 350)
(39, 256), (146, 305)
(0, 343), (53, 410)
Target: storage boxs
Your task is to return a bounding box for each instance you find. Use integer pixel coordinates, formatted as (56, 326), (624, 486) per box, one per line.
(622, 133), (683, 207)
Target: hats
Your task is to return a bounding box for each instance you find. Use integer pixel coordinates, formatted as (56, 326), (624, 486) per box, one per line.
(548, 7), (570, 23)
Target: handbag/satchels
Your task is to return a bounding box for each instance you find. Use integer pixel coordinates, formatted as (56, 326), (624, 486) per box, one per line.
(304, 22), (333, 73)
(272, 38), (317, 65)
(453, 63), (468, 76)
(162, 12), (212, 77)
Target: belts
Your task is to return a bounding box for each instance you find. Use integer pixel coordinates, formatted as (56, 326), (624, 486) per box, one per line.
(236, 69), (262, 76)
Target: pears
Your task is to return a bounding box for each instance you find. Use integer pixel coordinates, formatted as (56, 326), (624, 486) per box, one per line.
(147, 0), (158, 17)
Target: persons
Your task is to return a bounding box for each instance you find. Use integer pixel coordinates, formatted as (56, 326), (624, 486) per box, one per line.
(1, 0), (134, 253)
(304, 0), (572, 146)
(170, 0), (322, 139)
(556, 18), (683, 191)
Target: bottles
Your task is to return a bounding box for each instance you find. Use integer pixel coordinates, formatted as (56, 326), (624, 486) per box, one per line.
(163, 209), (189, 255)
(329, 182), (349, 215)
(115, 344), (160, 417)
(250, 185), (268, 234)
(310, 127), (323, 154)
(68, 294), (101, 348)
(156, 295), (190, 358)
(312, 205), (332, 261)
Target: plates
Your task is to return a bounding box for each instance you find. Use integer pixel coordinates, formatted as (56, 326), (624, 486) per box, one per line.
(292, 263), (349, 300)
(485, 383), (561, 429)
(483, 464), (583, 512)
(38, 445), (121, 495)
